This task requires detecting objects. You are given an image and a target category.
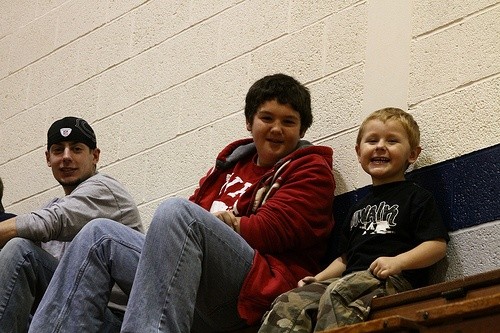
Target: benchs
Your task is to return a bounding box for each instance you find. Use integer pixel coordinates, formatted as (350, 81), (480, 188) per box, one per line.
(315, 269), (500, 333)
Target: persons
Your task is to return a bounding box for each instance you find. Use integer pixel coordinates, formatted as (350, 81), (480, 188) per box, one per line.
(258, 107), (450, 333)
(0, 116), (144, 333)
(27, 74), (337, 333)
(0, 177), (17, 225)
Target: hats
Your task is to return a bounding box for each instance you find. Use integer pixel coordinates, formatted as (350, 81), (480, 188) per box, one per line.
(47, 116), (97, 152)
